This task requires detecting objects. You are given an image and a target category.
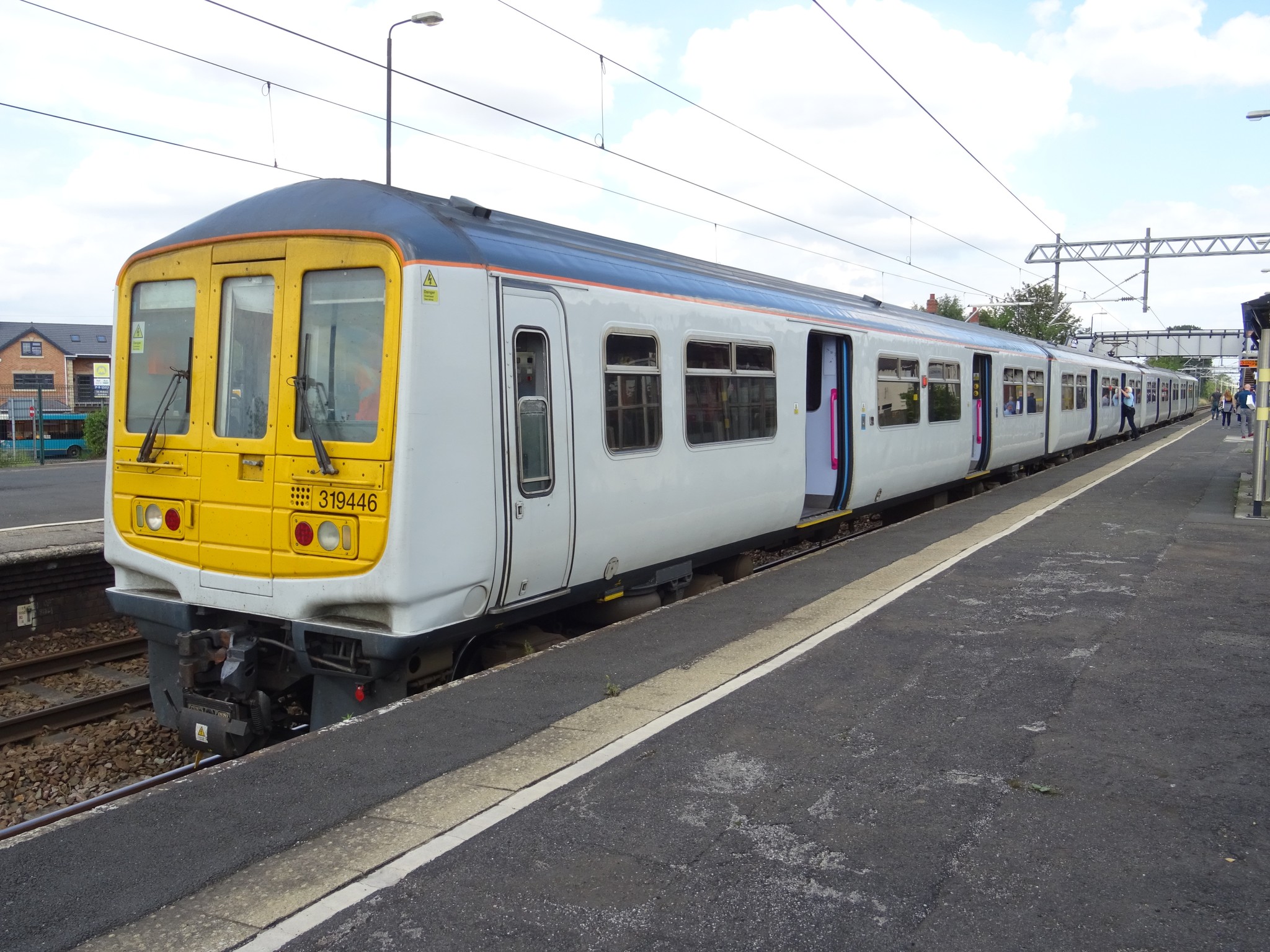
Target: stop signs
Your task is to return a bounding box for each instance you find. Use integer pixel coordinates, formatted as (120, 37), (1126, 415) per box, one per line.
(29, 406), (35, 417)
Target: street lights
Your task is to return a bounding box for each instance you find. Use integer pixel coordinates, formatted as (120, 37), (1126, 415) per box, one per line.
(384, 8), (443, 185)
(1091, 312), (1107, 337)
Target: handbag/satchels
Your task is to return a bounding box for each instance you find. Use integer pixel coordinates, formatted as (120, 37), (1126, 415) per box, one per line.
(1016, 401), (1020, 414)
(1218, 395), (1225, 410)
(1122, 407), (1135, 417)
(1246, 394), (1255, 410)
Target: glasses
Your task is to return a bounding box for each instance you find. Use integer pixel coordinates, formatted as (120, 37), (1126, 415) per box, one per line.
(1123, 388), (1128, 390)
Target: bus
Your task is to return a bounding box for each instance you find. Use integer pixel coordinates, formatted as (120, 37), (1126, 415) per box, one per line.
(0, 408), (96, 459)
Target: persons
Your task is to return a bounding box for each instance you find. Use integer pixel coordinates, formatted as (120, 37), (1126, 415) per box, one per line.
(1218, 389), (1237, 429)
(1234, 385), (1257, 426)
(1249, 368), (1256, 379)
(1017, 390), (1023, 414)
(1027, 391), (1036, 413)
(1004, 405), (1012, 416)
(1113, 388), (1119, 406)
(1238, 384), (1256, 439)
(1252, 369), (1257, 385)
(1005, 396), (1016, 415)
(1210, 388), (1222, 420)
(1102, 391), (1109, 407)
(1119, 386), (1141, 441)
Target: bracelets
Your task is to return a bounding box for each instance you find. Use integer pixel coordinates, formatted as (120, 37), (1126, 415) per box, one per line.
(1233, 407), (1235, 408)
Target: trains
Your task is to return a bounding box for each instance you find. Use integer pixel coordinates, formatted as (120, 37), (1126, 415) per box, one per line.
(104, 177), (1199, 751)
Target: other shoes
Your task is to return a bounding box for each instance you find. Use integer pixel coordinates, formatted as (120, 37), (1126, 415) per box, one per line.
(1221, 426), (1224, 428)
(1227, 426), (1231, 429)
(1239, 423), (1241, 425)
(1241, 436), (1245, 439)
(1216, 418), (1218, 420)
(1212, 415), (1214, 419)
(1134, 436), (1141, 440)
(1248, 433), (1254, 437)
